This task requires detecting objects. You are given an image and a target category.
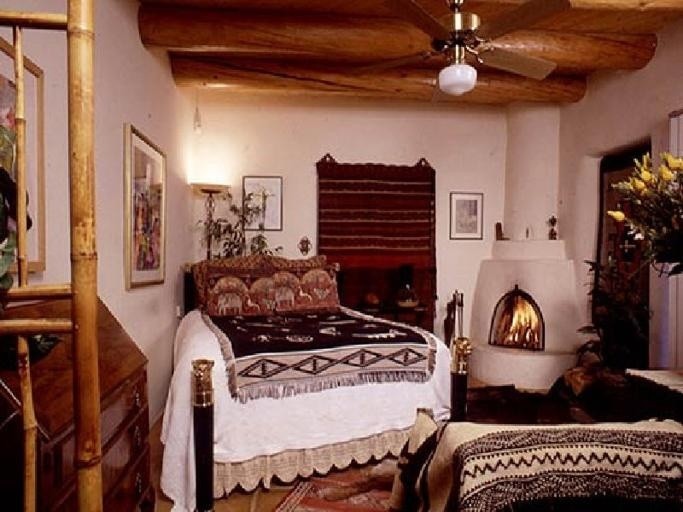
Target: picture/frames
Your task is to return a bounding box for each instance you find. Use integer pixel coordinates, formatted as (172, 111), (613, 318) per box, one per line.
(449, 190), (484, 240)
(123, 120), (168, 291)
(241, 176), (283, 232)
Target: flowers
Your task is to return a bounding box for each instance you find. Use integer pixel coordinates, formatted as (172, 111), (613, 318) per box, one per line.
(605, 150), (682, 282)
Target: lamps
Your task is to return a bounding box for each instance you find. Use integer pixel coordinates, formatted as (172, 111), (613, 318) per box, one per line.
(189, 182), (231, 260)
(437, 45), (477, 96)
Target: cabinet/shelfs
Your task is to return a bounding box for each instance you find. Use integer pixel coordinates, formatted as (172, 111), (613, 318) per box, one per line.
(0, 296), (155, 511)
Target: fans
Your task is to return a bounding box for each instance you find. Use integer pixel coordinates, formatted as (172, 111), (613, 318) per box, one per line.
(348, 0), (571, 82)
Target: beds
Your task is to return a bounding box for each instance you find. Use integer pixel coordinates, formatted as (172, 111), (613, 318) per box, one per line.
(159, 253), (474, 512)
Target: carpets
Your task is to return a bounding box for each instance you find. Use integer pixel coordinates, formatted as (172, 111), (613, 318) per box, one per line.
(270, 456), (391, 512)
